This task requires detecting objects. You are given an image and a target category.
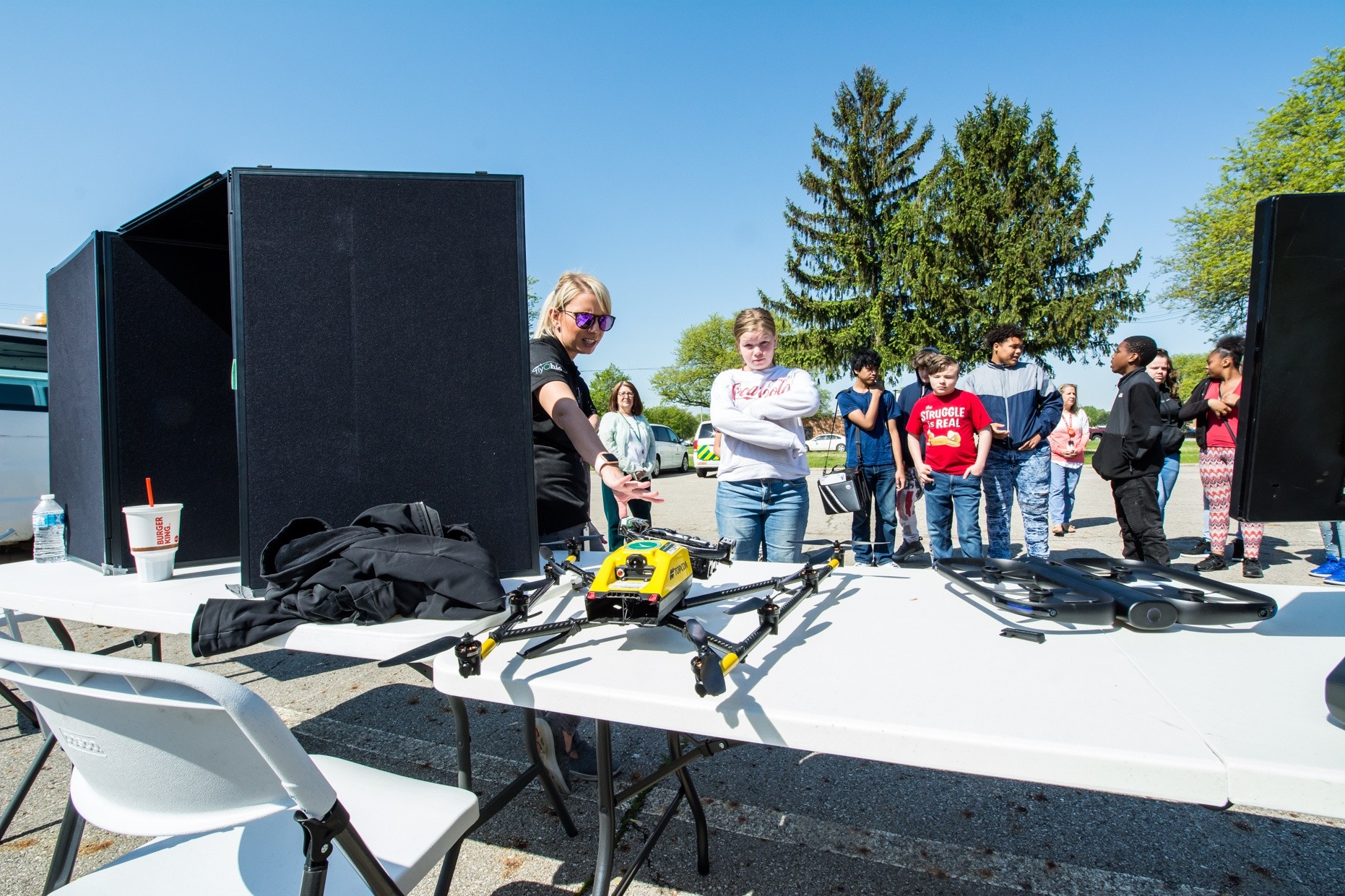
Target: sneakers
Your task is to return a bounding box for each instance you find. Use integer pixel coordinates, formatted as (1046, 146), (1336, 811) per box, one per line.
(874, 558), (901, 569)
(1194, 553), (1229, 572)
(1232, 539), (1245, 561)
(852, 555), (875, 567)
(1323, 558), (1345, 586)
(565, 731), (621, 781)
(534, 715), (572, 796)
(1180, 537), (1210, 558)
(892, 537), (924, 561)
(1309, 554), (1341, 577)
(1243, 558), (1264, 577)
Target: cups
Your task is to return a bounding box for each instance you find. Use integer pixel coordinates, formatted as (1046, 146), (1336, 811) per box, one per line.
(32, 494), (65, 563)
(122, 503), (184, 582)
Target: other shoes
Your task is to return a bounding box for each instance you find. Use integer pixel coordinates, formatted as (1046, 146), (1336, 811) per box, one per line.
(1053, 525), (1063, 537)
(1062, 523), (1075, 532)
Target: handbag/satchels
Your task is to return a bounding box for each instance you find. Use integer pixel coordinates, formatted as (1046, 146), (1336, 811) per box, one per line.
(818, 465), (867, 515)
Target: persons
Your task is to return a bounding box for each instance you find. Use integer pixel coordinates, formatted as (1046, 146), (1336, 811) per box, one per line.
(529, 273), (664, 552)
(1092, 335), (1345, 585)
(602, 380), (656, 551)
(835, 324), (1090, 568)
(710, 308), (819, 563)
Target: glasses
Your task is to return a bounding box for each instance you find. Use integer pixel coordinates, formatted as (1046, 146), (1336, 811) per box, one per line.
(618, 392), (635, 397)
(559, 310), (616, 331)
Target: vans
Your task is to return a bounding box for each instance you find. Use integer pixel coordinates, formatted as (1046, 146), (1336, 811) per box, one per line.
(692, 420), (721, 477)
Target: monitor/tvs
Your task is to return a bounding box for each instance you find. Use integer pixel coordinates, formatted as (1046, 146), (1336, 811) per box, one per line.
(1227, 191), (1345, 524)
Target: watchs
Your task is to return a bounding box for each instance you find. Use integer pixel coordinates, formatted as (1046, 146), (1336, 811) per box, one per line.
(594, 453), (618, 478)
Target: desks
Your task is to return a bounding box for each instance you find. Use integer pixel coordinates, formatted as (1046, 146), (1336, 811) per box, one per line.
(0, 543), (611, 896)
(429, 554), (1345, 896)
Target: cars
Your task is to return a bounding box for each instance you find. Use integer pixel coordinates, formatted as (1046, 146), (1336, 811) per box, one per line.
(649, 423), (689, 479)
(681, 440), (694, 447)
(805, 433), (846, 452)
(0, 314), (50, 548)
(1183, 427), (1196, 433)
(1088, 417), (1107, 441)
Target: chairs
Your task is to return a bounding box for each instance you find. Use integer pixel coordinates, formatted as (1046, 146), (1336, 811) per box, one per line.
(0, 642), (481, 896)
